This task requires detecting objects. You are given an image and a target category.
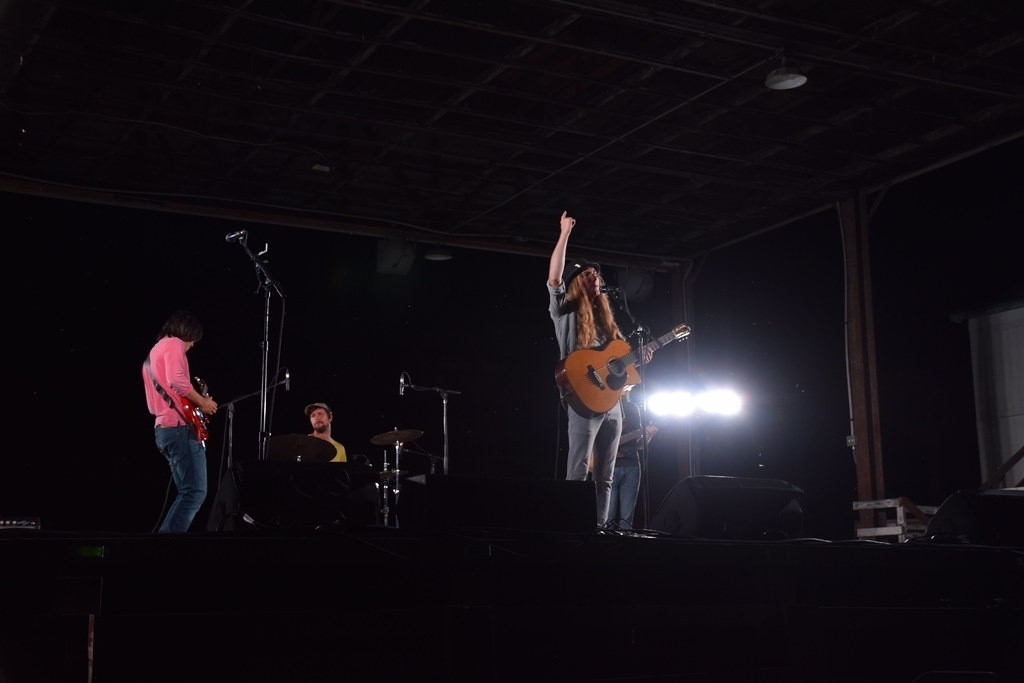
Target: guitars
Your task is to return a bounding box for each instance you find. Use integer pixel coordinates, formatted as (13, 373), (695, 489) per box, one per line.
(555, 323), (691, 420)
(183, 376), (208, 441)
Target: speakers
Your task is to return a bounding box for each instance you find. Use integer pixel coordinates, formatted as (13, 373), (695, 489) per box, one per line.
(228, 461), (375, 533)
(927, 489), (1024, 545)
(452, 481), (600, 536)
(646, 476), (803, 542)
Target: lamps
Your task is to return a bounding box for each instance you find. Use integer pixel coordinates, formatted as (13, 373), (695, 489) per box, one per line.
(765, 51), (810, 91)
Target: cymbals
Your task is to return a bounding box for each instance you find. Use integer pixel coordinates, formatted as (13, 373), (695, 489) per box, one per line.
(263, 434), (337, 463)
(370, 428), (423, 445)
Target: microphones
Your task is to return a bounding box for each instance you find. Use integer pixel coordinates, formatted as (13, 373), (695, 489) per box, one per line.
(599, 285), (623, 294)
(225, 229), (246, 243)
(285, 369), (290, 391)
(399, 374), (405, 396)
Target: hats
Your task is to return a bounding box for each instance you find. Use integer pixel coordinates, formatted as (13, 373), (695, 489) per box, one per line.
(305, 402), (331, 415)
(562, 260), (600, 288)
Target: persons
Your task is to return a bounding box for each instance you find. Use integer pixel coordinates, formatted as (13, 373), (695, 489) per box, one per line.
(546, 212), (654, 538)
(142, 313), (218, 533)
(303, 402), (348, 463)
(609, 386), (657, 533)
